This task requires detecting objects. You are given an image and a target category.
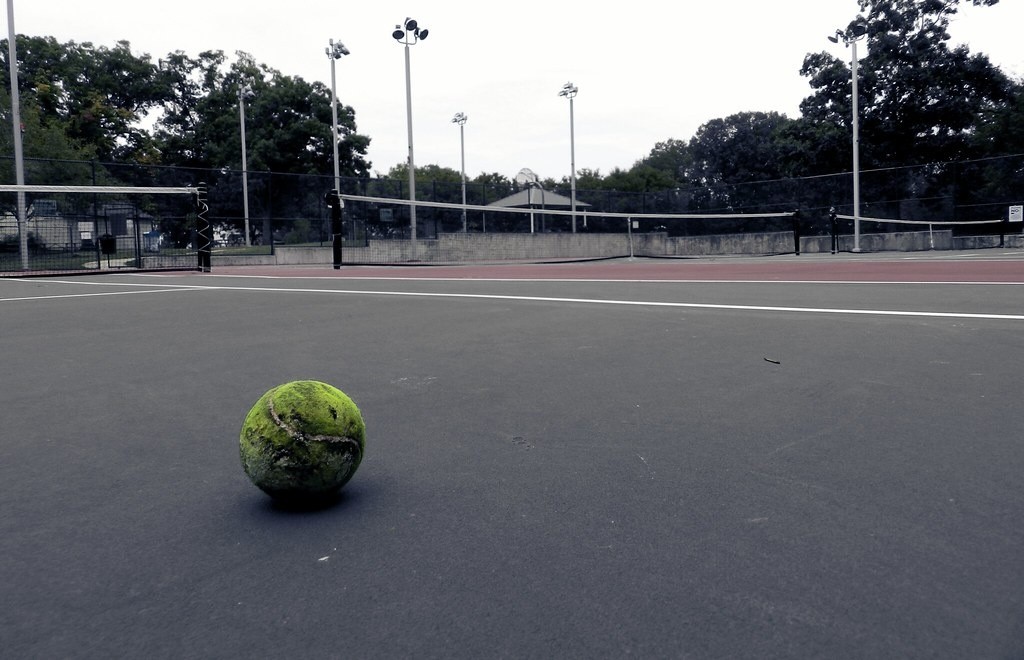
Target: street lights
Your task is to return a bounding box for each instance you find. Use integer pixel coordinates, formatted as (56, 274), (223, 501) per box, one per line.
(451, 108), (469, 235)
(236, 82), (255, 244)
(323, 39), (349, 270)
(391, 16), (428, 253)
(559, 80), (579, 236)
(850, 17), (870, 253)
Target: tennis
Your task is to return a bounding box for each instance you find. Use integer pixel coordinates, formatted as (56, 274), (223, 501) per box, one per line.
(236, 377), (368, 504)
